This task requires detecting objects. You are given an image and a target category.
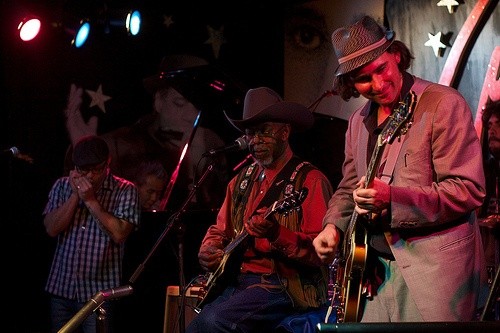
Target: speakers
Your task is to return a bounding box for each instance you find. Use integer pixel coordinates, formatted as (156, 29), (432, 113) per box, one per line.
(163, 285), (206, 333)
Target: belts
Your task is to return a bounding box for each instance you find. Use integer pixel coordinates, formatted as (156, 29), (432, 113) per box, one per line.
(373, 249), (395, 261)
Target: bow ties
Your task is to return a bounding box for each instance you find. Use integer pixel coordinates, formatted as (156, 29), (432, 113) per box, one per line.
(154, 127), (183, 141)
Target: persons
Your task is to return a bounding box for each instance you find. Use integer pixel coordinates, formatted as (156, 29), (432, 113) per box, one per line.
(43, 137), (139, 333)
(184, 86), (337, 332)
(313, 15), (486, 323)
(66, 71), (224, 208)
(134, 163), (168, 212)
(479, 99), (500, 216)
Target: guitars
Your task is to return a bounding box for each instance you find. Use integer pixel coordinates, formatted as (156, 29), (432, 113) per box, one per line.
(194, 187), (310, 308)
(324, 90), (416, 323)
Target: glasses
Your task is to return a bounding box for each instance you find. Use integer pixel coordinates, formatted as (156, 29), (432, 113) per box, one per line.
(242, 124), (287, 143)
(80, 162), (106, 176)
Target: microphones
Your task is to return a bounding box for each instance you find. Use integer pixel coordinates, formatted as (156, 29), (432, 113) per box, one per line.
(202, 137), (248, 158)
(0, 147), (20, 160)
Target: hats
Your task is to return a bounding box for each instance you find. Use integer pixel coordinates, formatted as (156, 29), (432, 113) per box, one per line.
(75, 136), (108, 166)
(332, 16), (396, 76)
(236, 87), (314, 132)
(165, 66), (216, 112)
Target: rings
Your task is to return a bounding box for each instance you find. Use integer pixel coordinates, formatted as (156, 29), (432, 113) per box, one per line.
(65, 109), (71, 117)
(77, 185), (81, 189)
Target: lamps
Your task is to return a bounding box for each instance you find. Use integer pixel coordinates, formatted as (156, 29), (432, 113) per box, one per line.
(9, 2), (143, 50)
